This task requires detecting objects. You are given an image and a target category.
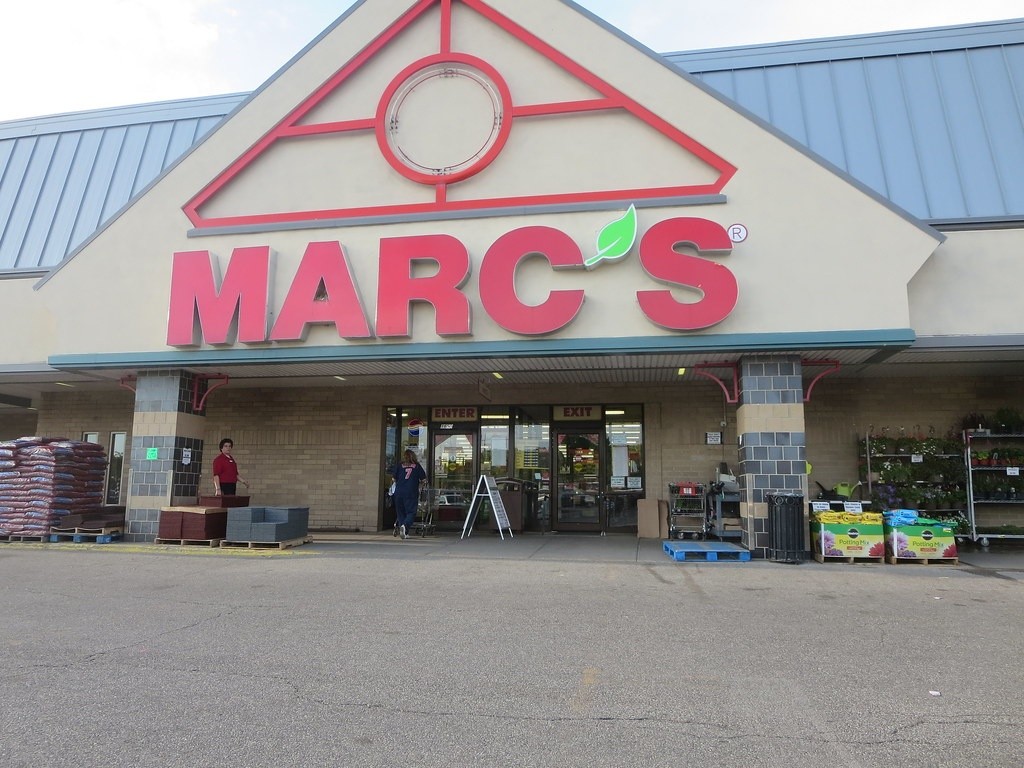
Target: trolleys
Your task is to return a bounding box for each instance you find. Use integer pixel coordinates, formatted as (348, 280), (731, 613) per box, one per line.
(668, 482), (708, 539)
(392, 480), (437, 538)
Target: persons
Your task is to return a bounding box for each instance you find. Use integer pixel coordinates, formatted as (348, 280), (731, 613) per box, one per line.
(392, 449), (427, 539)
(212, 438), (249, 496)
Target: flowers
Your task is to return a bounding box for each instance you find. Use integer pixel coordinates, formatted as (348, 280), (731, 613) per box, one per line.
(868, 418), (963, 508)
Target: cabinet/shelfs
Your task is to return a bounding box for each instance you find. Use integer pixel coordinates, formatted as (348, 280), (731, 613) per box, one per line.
(666, 486), (707, 535)
(964, 429), (1024, 540)
(708, 495), (743, 541)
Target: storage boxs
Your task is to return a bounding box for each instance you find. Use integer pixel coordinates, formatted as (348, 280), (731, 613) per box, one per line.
(680, 488), (696, 495)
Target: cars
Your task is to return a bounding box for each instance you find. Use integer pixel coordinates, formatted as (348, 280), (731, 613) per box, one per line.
(486, 477), (539, 493)
(445, 480), (485, 512)
(424, 495), (470, 506)
(561, 492), (600, 518)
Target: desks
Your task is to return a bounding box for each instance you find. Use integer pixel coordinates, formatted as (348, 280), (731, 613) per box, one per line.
(809, 499), (871, 513)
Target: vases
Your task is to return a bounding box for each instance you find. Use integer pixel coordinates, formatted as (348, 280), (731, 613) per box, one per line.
(867, 446), (964, 509)
(859, 429), (974, 545)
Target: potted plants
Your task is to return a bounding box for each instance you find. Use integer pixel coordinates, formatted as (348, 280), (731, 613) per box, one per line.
(964, 408), (1024, 499)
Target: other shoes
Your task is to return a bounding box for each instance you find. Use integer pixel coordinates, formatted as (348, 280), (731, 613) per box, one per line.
(405, 534), (411, 539)
(400, 524), (406, 539)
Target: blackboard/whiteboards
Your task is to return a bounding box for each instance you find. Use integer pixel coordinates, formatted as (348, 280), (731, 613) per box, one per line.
(463, 475), (511, 528)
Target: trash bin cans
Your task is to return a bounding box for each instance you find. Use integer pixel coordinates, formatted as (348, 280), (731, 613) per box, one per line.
(766, 493), (806, 563)
